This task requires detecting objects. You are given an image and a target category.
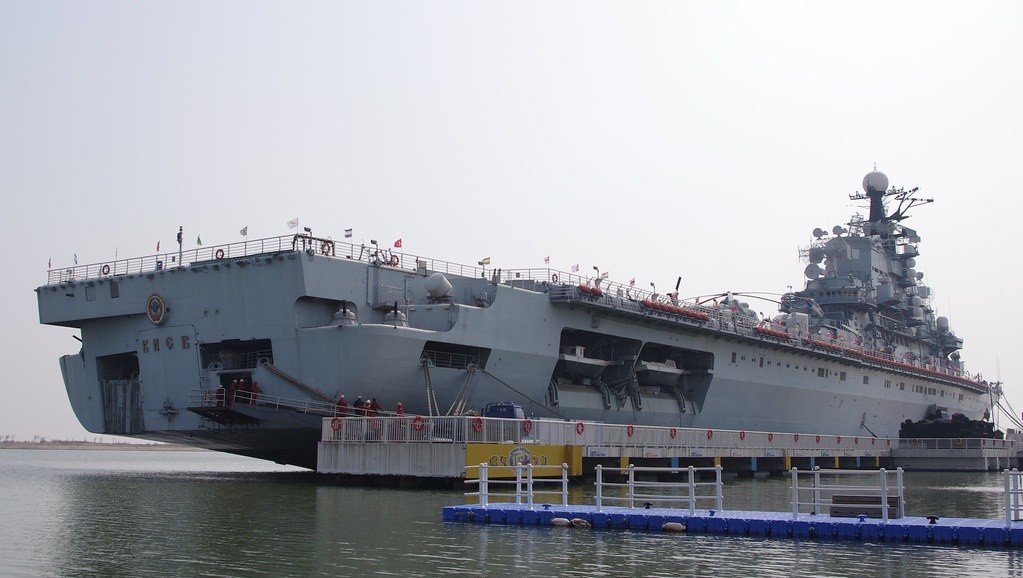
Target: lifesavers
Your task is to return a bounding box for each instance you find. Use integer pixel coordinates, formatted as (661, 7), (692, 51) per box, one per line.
(524, 419), (532, 432)
(816, 435), (821, 443)
(740, 432), (745, 440)
(794, 433), (798, 442)
(708, 430), (713, 439)
(872, 438), (875, 444)
(955, 438), (962, 445)
(576, 422), (584, 435)
(551, 273), (558, 283)
(671, 429), (676, 438)
(887, 440), (890, 445)
(855, 437), (858, 443)
(321, 243), (330, 253)
(837, 437), (841, 444)
(413, 417), (424, 430)
(216, 249), (224, 259)
(473, 418), (482, 432)
(331, 418), (341, 430)
(911, 438), (918, 445)
(391, 255), (399, 265)
(768, 433), (773, 441)
(102, 264), (110, 275)
(628, 425), (634, 437)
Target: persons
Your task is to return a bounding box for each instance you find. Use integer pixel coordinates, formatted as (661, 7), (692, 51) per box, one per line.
(336, 395), (348, 417)
(216, 385), (224, 406)
(250, 382), (263, 406)
(229, 379), (245, 402)
(396, 403), (403, 416)
(860, 340), (864, 347)
(353, 396), (384, 417)
(795, 322), (800, 336)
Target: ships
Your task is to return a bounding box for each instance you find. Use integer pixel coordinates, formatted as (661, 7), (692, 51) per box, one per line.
(37, 167), (1002, 477)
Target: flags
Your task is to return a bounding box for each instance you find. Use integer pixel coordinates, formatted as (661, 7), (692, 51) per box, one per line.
(544, 257), (549, 264)
(197, 236), (201, 245)
(602, 272), (608, 278)
(394, 239), (401, 247)
(571, 264), (578, 273)
(696, 297), (699, 303)
(629, 278), (635, 287)
(48, 258), (51, 268)
(240, 226), (247, 236)
(157, 242), (159, 251)
(74, 252), (77, 265)
(478, 257), (490, 265)
(287, 216), (298, 230)
(344, 229), (352, 238)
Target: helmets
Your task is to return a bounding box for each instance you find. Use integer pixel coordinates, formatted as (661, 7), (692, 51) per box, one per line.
(253, 381), (257, 384)
(358, 396), (361, 398)
(366, 400), (370, 403)
(340, 395), (344, 398)
(233, 380), (237, 382)
(219, 385), (222, 387)
(240, 379), (244, 382)
